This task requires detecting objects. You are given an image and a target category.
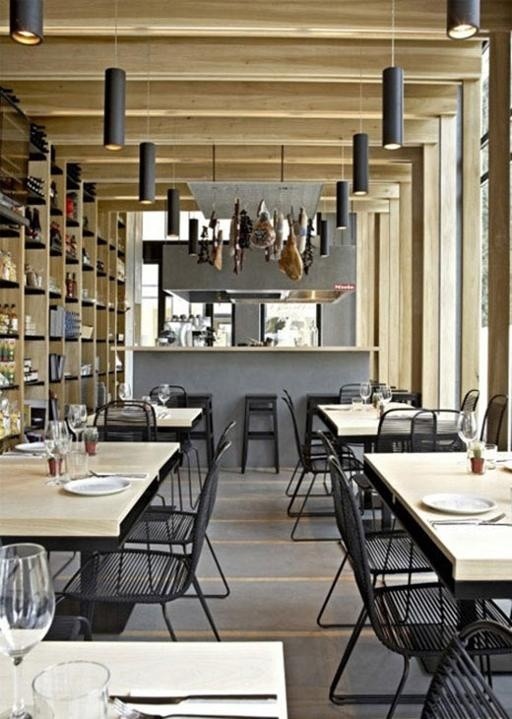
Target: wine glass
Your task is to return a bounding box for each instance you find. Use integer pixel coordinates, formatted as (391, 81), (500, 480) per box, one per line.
(459, 410), (478, 463)
(359, 381), (393, 415)
(0, 542), (57, 719)
(119, 382), (171, 413)
(46, 403), (90, 488)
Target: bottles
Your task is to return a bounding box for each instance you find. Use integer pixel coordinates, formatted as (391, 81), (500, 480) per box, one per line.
(305, 318), (318, 348)
(67, 272), (77, 298)
(50, 221), (62, 250)
(27, 207), (46, 241)
(464, 440), (487, 474)
(0, 302), (20, 439)
(65, 232), (78, 258)
(84, 427), (99, 458)
(67, 194), (74, 219)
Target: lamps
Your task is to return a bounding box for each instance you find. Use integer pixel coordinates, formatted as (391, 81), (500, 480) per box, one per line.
(447, 0), (480, 40)
(188, 195), (198, 257)
(320, 186), (329, 259)
(167, 146), (180, 237)
(103, 0), (126, 151)
(335, 146), (349, 230)
(9, 0), (44, 46)
(381, 0), (405, 149)
(352, 84), (368, 196)
(138, 80), (155, 204)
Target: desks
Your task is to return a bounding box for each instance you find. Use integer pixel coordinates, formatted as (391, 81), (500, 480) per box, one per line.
(0, 403), (512, 719)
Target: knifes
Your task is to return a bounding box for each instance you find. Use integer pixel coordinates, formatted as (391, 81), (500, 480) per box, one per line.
(111, 690), (280, 706)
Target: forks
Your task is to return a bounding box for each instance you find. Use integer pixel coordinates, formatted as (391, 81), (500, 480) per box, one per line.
(112, 698), (284, 718)
(88, 469), (148, 479)
(429, 512), (507, 525)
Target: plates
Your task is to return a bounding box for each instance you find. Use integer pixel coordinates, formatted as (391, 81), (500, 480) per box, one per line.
(14, 442), (46, 454)
(423, 492), (495, 516)
(63, 478), (131, 495)
(325, 404), (351, 411)
(494, 451), (512, 471)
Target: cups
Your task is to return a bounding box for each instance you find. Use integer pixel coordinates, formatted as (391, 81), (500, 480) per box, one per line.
(352, 397), (363, 412)
(480, 444), (500, 470)
(31, 661), (115, 718)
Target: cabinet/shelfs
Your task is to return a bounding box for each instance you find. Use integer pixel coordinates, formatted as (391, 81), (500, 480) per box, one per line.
(0, 91), (127, 452)
